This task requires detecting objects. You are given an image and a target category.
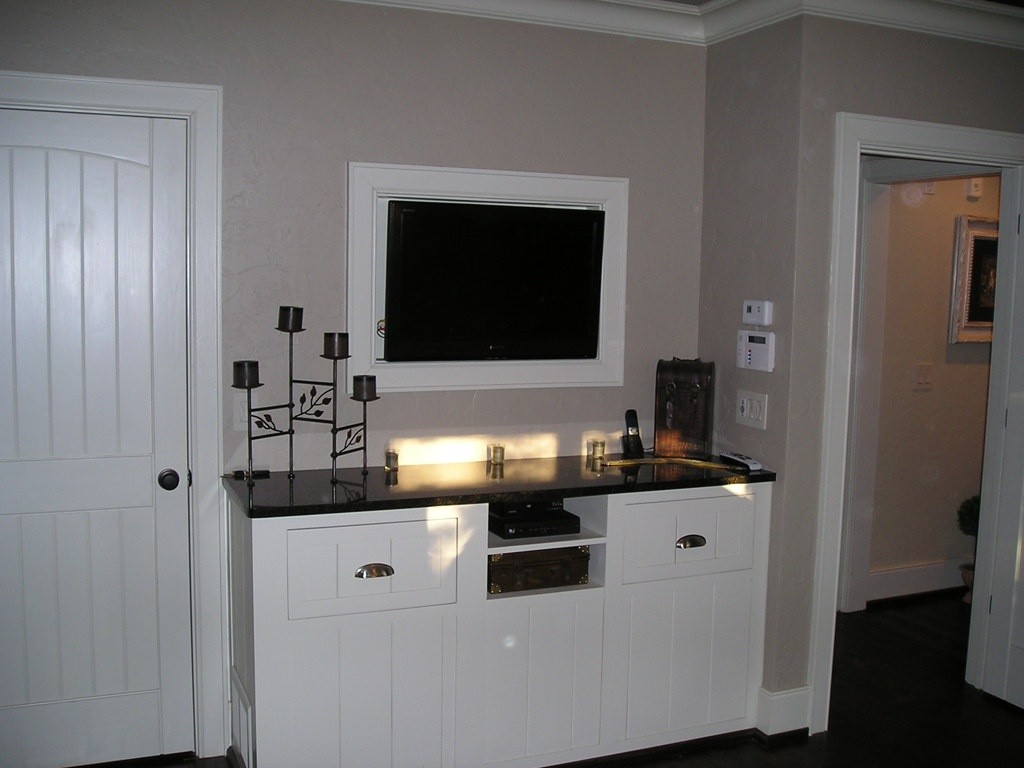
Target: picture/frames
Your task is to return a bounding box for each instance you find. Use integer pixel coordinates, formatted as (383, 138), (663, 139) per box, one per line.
(947, 213), (999, 345)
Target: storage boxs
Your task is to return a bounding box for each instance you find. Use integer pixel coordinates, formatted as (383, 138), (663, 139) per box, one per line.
(487, 545), (591, 594)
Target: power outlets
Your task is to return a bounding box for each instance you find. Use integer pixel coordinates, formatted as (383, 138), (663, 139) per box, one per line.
(924, 182), (936, 195)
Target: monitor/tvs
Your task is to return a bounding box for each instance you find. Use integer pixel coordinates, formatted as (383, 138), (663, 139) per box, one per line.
(384, 199), (605, 359)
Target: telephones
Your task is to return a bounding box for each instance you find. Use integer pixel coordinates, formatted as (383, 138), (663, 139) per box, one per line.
(621, 408), (645, 460)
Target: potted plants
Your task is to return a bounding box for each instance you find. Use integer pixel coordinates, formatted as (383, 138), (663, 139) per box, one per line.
(956, 495), (980, 603)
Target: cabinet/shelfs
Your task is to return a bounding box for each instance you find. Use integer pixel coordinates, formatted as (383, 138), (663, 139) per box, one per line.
(222, 450), (776, 768)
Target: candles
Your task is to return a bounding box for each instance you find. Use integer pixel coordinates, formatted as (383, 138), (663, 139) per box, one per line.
(233, 360), (259, 387)
(352, 375), (377, 401)
(592, 438), (606, 459)
(278, 305), (303, 331)
(384, 448), (399, 472)
(324, 331), (349, 357)
(490, 442), (506, 465)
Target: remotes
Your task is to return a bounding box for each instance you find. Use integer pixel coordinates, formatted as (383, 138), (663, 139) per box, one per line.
(720, 452), (761, 471)
